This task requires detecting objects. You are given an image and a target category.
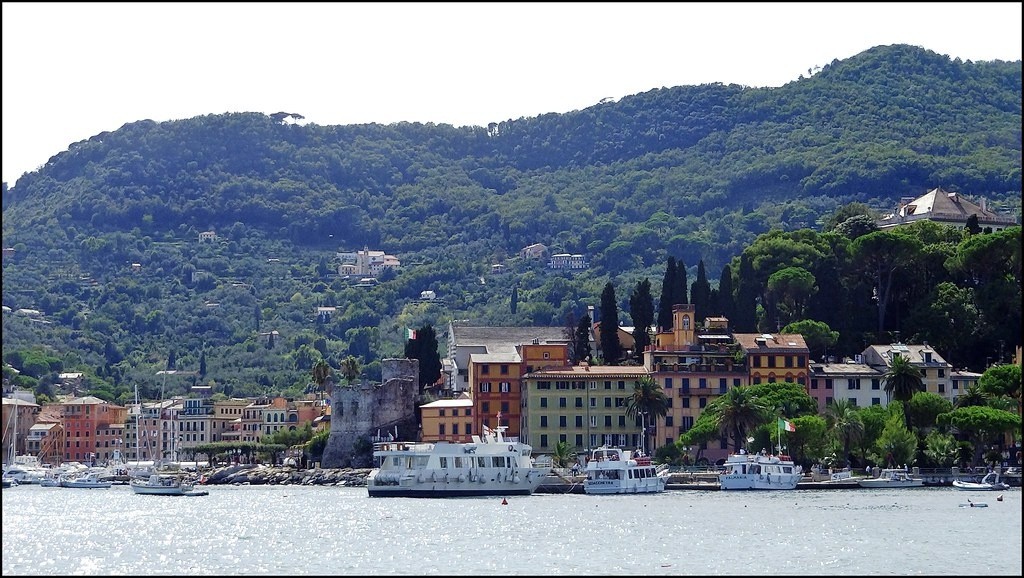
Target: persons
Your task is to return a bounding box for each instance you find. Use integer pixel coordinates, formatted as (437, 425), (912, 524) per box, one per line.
(721, 444), (803, 484)
(598, 447), (658, 480)
(573, 462), (580, 475)
(811, 465), (908, 480)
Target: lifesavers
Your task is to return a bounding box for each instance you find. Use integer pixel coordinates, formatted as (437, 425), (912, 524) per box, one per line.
(508, 445), (513, 451)
(397, 443), (405, 451)
(381, 444), (390, 451)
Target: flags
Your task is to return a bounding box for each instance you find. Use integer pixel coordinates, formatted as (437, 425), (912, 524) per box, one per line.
(483, 424), (496, 436)
(778, 417), (796, 432)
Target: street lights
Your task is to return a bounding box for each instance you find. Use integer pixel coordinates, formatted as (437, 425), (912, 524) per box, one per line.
(638, 412), (645, 458)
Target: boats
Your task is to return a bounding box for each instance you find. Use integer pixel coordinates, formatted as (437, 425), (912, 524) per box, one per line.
(951, 471), (1010, 491)
(3, 361), (195, 497)
(855, 464), (925, 488)
(718, 448), (805, 490)
(581, 444), (673, 495)
(366, 412), (555, 497)
